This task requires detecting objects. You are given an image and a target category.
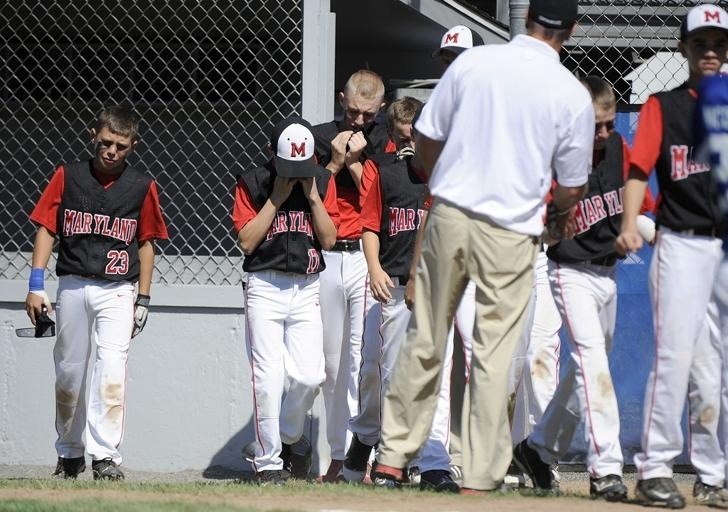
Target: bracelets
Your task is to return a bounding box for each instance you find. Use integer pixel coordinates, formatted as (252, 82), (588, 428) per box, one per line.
(135, 294), (150, 308)
(29, 268), (45, 291)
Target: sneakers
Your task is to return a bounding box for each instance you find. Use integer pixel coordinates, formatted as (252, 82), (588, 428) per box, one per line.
(288, 434), (313, 481)
(255, 469), (283, 487)
(418, 469), (460, 494)
(634, 477), (685, 509)
(370, 457), (411, 489)
(342, 432), (373, 484)
(91, 456), (125, 482)
(589, 473), (628, 502)
(50, 455), (87, 482)
(512, 437), (561, 497)
(692, 480), (728, 508)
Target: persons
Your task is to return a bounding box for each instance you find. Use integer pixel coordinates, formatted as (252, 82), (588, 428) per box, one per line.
(25, 107), (168, 483)
(232, 1), (728, 508)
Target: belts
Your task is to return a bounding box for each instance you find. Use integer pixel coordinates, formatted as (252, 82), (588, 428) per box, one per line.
(671, 227), (724, 239)
(329, 240), (361, 252)
(390, 276), (409, 286)
(585, 255), (617, 268)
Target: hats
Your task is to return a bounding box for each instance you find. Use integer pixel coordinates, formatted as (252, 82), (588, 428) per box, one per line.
(270, 115), (316, 179)
(527, 0), (579, 30)
(680, 3), (728, 40)
(431, 24), (485, 60)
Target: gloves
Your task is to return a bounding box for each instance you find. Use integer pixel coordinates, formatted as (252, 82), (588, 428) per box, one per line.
(131, 293), (151, 340)
